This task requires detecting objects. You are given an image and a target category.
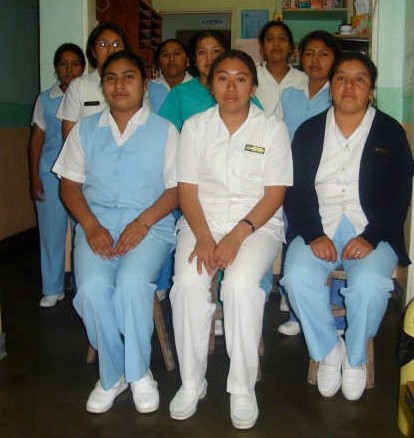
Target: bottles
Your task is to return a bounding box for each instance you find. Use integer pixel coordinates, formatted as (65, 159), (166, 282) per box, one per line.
(340, 24), (352, 36)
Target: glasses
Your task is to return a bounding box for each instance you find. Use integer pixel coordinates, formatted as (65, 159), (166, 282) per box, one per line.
(94, 39), (123, 50)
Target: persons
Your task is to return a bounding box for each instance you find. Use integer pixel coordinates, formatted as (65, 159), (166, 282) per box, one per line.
(157, 30), (262, 337)
(48, 53), (183, 415)
(255, 20), (309, 292)
(159, 50), (293, 429)
(144, 39), (195, 298)
(288, 54), (412, 405)
(278, 30), (344, 335)
(56, 22), (134, 146)
(30, 41), (87, 306)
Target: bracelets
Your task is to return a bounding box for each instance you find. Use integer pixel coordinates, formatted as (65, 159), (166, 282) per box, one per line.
(241, 218), (256, 232)
(135, 217), (150, 231)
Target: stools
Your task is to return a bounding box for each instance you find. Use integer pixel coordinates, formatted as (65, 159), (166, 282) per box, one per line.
(82, 262), (381, 388)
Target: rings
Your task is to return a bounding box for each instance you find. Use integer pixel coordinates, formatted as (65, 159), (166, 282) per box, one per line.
(356, 250), (361, 255)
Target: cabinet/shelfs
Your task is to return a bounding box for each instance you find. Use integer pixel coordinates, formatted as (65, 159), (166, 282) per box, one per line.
(97, 0), (165, 79)
(282, 0), (354, 69)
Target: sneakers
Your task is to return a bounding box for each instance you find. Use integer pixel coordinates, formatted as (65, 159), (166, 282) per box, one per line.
(318, 340), (345, 397)
(130, 371), (160, 414)
(231, 389), (259, 430)
(278, 320), (301, 335)
(169, 380), (207, 420)
(214, 319), (223, 335)
(155, 290), (167, 301)
(336, 330), (345, 335)
(40, 293), (65, 307)
(342, 355), (367, 402)
(85, 375), (128, 414)
